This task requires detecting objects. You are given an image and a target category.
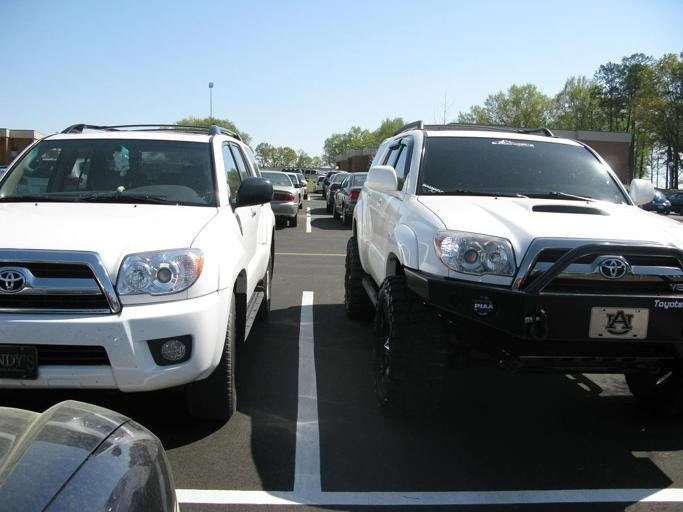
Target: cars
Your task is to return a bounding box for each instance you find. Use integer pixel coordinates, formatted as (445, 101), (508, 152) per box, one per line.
(0, 399), (179, 512)
(640, 188), (683, 215)
(258, 169), (368, 227)
(343, 120), (683, 417)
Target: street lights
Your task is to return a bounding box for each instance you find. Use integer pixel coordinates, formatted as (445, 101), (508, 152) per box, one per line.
(207, 80), (214, 119)
